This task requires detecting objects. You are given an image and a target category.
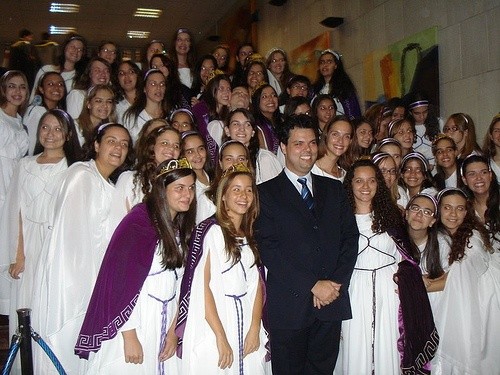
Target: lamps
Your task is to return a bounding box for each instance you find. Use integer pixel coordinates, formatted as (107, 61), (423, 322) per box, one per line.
(209, 36), (221, 42)
(318, 16), (344, 29)
(268, 0), (287, 6)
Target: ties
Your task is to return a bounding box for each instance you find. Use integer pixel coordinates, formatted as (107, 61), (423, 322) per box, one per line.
(297, 178), (313, 210)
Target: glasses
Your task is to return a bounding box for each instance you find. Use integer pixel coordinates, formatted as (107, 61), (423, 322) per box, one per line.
(152, 64), (163, 69)
(248, 72), (263, 77)
(404, 168), (423, 174)
(104, 49), (116, 56)
(441, 205), (465, 214)
(172, 122), (192, 129)
(201, 66), (215, 71)
(272, 59), (285, 63)
(217, 87), (231, 91)
(320, 106), (335, 110)
(396, 129), (412, 136)
(149, 81), (166, 87)
(119, 68), (135, 76)
(292, 85), (307, 91)
(214, 53), (225, 60)
(381, 168), (399, 175)
(445, 127), (459, 133)
(232, 122), (251, 128)
(297, 112), (311, 116)
(409, 206), (435, 218)
(359, 129), (373, 136)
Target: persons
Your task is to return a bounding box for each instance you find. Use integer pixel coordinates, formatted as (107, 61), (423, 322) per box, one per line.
(180, 171), (264, 375)
(88, 158), (197, 374)
(0, 29), (500, 375)
(251, 114), (360, 375)
